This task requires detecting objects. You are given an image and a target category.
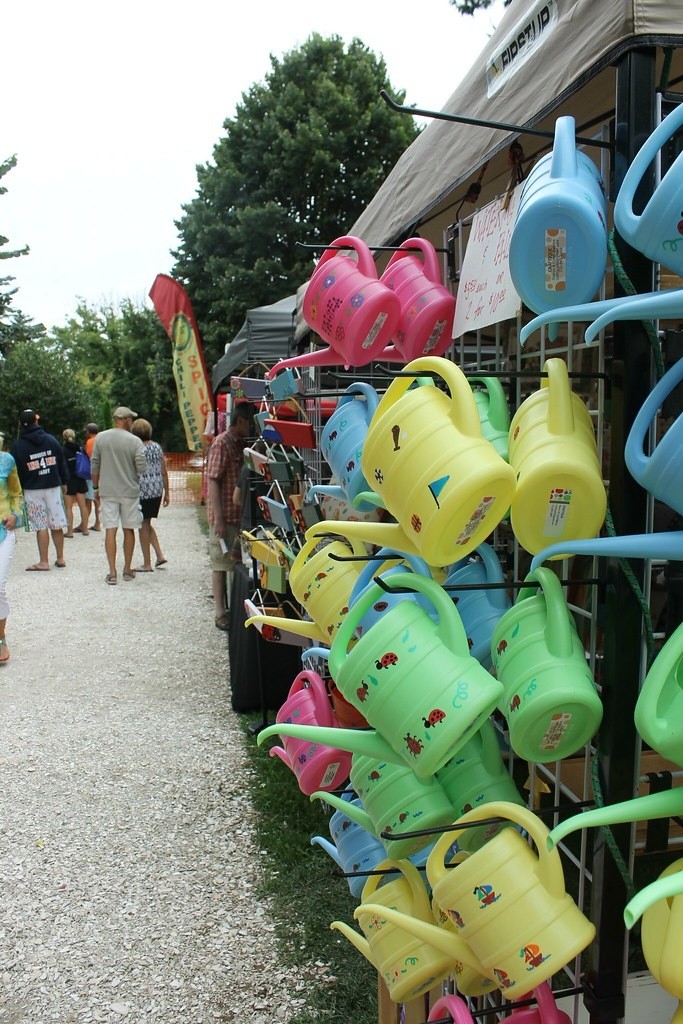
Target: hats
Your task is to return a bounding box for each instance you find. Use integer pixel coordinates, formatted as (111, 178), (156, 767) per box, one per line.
(113, 406), (138, 418)
(20, 409), (40, 428)
(83, 423), (99, 433)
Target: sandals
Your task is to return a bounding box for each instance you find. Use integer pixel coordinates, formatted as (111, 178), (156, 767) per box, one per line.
(105, 574), (118, 584)
(0, 638), (10, 661)
(122, 571), (135, 580)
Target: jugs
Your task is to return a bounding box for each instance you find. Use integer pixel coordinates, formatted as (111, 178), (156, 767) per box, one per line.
(244, 100), (683, 1024)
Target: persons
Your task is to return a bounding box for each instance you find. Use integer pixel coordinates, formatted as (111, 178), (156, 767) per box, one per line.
(132, 419), (170, 572)
(62, 429), (90, 538)
(207, 403), (271, 632)
(92, 408), (147, 585)
(14, 410), (70, 570)
(0, 431), (23, 661)
(73, 423), (100, 533)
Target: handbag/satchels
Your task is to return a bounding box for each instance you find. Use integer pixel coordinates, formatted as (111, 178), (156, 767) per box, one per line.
(75, 447), (91, 480)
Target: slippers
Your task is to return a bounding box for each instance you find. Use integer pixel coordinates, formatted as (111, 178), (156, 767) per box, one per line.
(131, 566), (153, 572)
(64, 533), (73, 538)
(73, 528), (83, 532)
(56, 560), (66, 567)
(89, 527), (98, 531)
(155, 560), (167, 567)
(24, 564), (49, 570)
(82, 531), (89, 536)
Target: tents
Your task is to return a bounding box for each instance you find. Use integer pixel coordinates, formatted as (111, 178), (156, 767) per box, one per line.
(212, 0), (683, 1024)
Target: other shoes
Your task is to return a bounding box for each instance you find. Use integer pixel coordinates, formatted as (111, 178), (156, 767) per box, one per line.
(215, 614), (230, 630)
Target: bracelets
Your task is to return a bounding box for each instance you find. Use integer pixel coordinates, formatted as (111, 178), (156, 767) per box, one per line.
(93, 486), (99, 492)
